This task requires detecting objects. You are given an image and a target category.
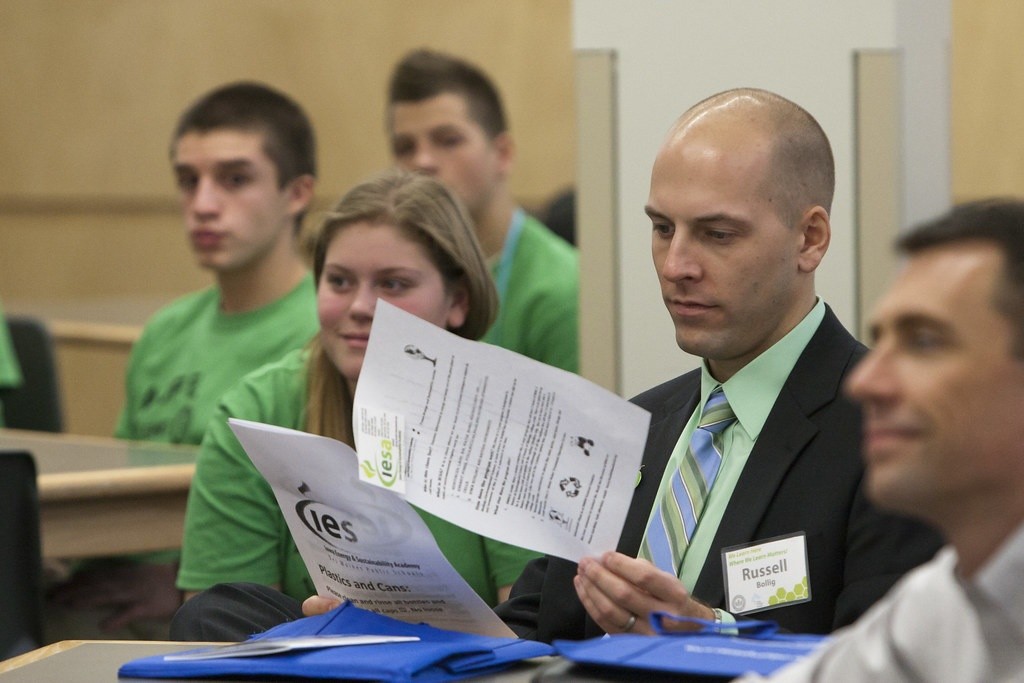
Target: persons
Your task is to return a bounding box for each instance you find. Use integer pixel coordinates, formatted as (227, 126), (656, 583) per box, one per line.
(42, 80), (322, 642)
(735, 196), (1024, 683)
(301, 86), (947, 658)
(168, 175), (547, 642)
(386, 49), (580, 377)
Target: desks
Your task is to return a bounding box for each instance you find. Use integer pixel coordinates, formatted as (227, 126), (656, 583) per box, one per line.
(0, 637), (571, 683)
(0, 427), (206, 559)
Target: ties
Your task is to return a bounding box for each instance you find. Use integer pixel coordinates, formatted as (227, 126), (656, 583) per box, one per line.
(636, 389), (739, 578)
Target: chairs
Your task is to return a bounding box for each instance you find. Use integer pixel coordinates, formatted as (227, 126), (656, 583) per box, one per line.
(0, 315), (67, 432)
(0, 450), (46, 662)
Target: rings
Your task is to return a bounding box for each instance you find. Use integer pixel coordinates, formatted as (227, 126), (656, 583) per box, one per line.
(620, 613), (637, 631)
